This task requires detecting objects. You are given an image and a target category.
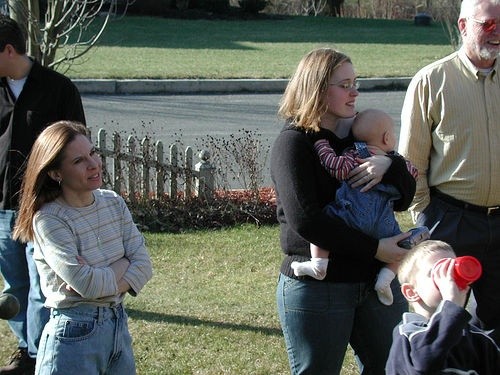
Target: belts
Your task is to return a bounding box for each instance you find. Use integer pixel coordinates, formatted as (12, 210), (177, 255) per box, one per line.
(430, 185), (500, 218)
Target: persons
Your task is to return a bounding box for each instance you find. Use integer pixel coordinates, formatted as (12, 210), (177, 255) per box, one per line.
(0, 13), (86, 375)
(269, 48), (416, 375)
(291, 108), (421, 305)
(385, 238), (500, 375)
(398, 0), (500, 334)
(12, 121), (152, 374)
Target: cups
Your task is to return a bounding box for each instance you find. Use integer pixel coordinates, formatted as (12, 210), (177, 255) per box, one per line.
(431, 256), (482, 291)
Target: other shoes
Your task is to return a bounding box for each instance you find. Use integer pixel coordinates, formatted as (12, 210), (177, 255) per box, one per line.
(0, 347), (35, 375)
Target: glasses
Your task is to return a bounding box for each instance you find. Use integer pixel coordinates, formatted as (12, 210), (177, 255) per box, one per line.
(467, 16), (500, 32)
(328, 81), (360, 91)
